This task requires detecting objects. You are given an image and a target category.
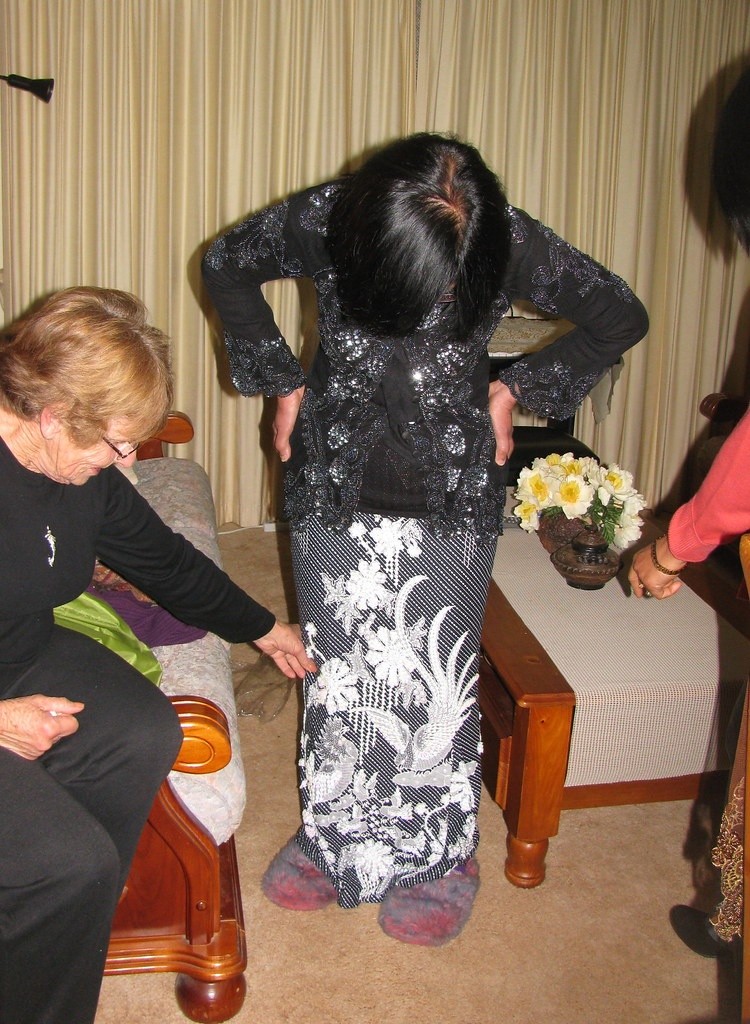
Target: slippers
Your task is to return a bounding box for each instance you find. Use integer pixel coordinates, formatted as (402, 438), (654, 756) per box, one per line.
(261, 834), (336, 911)
(378, 868), (482, 945)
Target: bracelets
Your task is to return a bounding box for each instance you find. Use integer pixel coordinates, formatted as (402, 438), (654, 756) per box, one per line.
(647, 537), (681, 576)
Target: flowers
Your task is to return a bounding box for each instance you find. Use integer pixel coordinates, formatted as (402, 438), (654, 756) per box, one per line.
(517, 452), (648, 549)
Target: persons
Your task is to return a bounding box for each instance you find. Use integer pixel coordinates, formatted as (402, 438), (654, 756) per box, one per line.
(628, 67), (750, 959)
(0, 285), (318, 1024)
(200, 132), (649, 947)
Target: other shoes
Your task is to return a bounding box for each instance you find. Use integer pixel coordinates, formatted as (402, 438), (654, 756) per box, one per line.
(670, 904), (742, 957)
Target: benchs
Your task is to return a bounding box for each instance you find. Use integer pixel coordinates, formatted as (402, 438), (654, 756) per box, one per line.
(692, 391), (750, 554)
(77, 412), (248, 1023)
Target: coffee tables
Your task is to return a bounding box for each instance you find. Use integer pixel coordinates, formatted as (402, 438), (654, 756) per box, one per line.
(482, 525), (750, 889)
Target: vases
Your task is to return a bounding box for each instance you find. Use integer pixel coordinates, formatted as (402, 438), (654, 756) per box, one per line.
(538, 508), (583, 552)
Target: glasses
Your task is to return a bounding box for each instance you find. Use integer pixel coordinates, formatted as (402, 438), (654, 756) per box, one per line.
(98, 432), (140, 459)
